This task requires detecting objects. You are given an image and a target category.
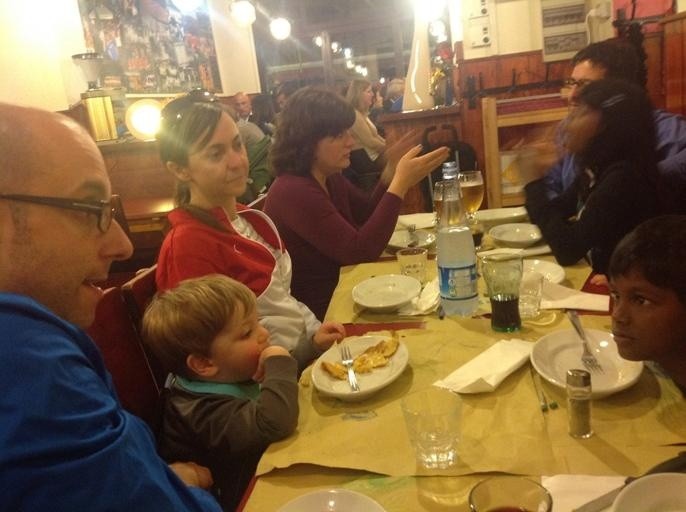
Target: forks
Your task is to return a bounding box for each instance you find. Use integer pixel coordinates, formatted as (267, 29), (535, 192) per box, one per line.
(566, 310), (606, 375)
(338, 346), (360, 391)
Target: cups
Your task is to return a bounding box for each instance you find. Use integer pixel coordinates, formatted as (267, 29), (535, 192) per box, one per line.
(481, 252), (524, 334)
(500, 150), (518, 183)
(468, 476), (553, 511)
(399, 387), (463, 468)
(434, 181), (465, 227)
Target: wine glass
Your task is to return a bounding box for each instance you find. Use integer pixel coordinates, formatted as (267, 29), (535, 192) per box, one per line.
(459, 170), (485, 225)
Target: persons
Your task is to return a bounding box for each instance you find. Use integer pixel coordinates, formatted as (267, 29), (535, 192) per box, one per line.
(0, 103), (225, 511)
(156, 88), (322, 353)
(212, 78), (406, 204)
(262, 88), (451, 323)
(607, 216), (684, 391)
(142, 276), (347, 512)
(525, 38), (684, 271)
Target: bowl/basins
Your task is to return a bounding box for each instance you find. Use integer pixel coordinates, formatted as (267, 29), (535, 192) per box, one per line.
(350, 207), (569, 315)
(530, 326), (645, 399)
(311, 334), (411, 402)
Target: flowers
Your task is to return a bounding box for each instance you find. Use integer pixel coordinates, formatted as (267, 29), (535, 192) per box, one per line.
(435, 44), (456, 77)
(426, 69), (446, 95)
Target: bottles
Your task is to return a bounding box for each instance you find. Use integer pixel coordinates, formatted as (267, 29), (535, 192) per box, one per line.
(435, 161), (480, 319)
(565, 368), (595, 438)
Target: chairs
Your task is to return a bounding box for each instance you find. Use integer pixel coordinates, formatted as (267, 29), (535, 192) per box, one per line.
(244, 193), (267, 212)
(84, 286), (159, 424)
(119, 259), (176, 391)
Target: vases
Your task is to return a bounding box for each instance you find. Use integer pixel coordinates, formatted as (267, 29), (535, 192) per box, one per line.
(444, 80), (454, 107)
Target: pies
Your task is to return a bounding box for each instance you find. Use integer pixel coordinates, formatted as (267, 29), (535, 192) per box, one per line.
(323, 335), (399, 381)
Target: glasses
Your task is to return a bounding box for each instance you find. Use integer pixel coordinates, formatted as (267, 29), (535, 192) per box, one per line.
(161, 88), (217, 123)
(1, 194), (124, 234)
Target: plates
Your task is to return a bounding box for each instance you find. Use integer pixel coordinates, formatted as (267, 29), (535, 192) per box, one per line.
(274, 488), (386, 512)
(610, 471), (686, 512)
